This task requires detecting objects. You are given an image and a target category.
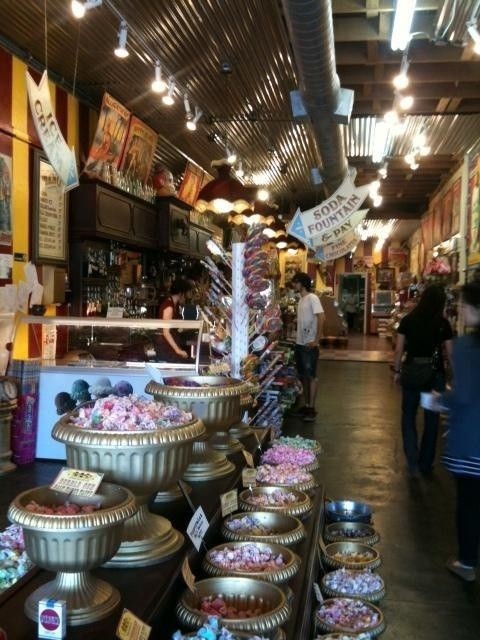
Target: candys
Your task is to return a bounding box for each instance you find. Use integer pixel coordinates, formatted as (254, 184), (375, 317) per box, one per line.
(0, 523), (37, 596)
(171, 435), (318, 640)
(199, 223), (302, 427)
(67, 394), (193, 432)
(25, 500), (101, 514)
(315, 528), (381, 640)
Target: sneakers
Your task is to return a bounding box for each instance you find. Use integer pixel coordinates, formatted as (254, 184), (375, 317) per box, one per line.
(447, 554), (476, 581)
(288, 406), (316, 422)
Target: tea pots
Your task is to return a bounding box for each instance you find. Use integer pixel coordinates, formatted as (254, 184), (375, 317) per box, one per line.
(135, 276), (156, 303)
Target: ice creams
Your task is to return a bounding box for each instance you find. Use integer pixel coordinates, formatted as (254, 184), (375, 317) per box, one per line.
(54, 376), (133, 414)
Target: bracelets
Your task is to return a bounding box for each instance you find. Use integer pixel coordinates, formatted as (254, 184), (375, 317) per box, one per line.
(390, 364), (401, 374)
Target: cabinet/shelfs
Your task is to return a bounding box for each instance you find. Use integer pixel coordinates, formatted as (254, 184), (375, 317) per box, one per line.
(1, 425), (387, 640)
(69, 177), (218, 359)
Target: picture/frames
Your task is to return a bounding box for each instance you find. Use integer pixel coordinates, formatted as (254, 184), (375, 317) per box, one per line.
(376, 267), (395, 283)
(29, 144), (70, 268)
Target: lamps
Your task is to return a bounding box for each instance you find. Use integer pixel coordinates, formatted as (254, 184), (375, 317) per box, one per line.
(262, 219), (306, 257)
(391, 0), (418, 54)
(361, 66), (432, 248)
(69, 0), (206, 133)
(223, 139), (272, 201)
(464, 19), (480, 58)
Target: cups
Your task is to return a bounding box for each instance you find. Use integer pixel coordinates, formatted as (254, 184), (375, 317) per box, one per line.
(419, 392), (449, 414)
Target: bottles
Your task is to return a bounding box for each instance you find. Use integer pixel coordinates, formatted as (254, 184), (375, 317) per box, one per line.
(85, 239), (148, 345)
(102, 164), (156, 204)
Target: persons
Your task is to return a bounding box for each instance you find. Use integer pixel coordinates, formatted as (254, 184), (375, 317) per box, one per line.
(392, 286), (454, 498)
(430, 284), (480, 583)
(340, 290), (358, 332)
(153, 278), (190, 363)
(291, 271), (327, 422)
